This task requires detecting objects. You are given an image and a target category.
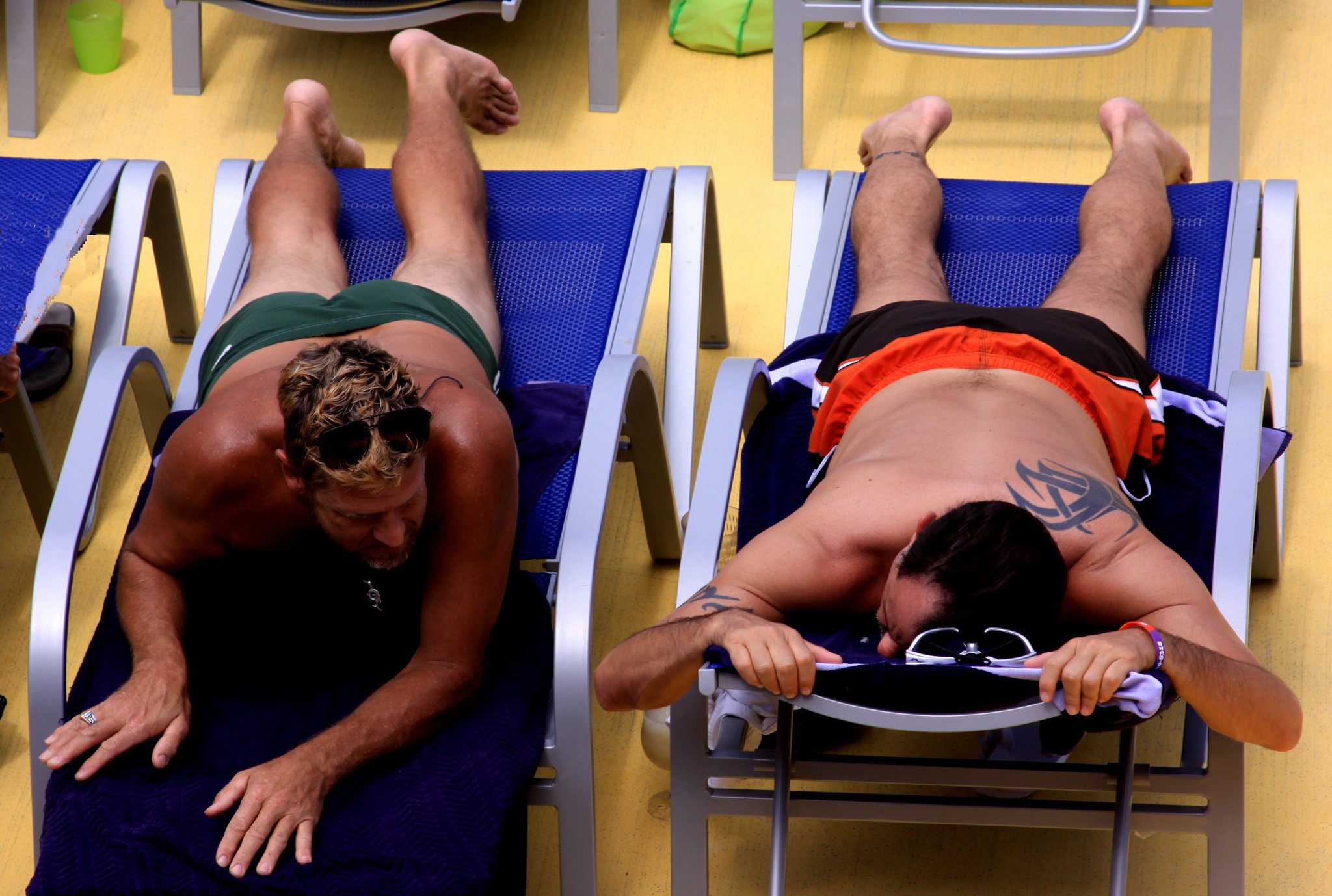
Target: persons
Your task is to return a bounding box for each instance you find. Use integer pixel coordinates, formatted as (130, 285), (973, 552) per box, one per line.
(594, 97), (1304, 753)
(40, 30), (520, 875)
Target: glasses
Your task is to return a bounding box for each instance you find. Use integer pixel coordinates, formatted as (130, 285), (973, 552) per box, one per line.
(906, 629), (1035, 668)
(290, 406), (432, 468)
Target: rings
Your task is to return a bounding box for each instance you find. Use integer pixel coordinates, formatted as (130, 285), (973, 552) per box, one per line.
(80, 711), (97, 726)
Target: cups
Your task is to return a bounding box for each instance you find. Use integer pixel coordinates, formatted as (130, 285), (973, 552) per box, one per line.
(65, 0), (122, 73)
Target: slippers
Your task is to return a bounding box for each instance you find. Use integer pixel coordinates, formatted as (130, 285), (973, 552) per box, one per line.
(21, 301), (75, 399)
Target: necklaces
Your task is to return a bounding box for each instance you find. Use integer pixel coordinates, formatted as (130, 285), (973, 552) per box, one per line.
(363, 579), (382, 611)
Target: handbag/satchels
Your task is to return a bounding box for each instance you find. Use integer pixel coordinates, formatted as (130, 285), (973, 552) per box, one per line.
(666, 2), (828, 53)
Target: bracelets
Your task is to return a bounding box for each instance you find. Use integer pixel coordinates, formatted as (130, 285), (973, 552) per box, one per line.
(1118, 622), (1165, 670)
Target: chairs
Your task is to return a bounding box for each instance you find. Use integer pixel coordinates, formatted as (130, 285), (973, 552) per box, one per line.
(0, 0), (1302, 896)
